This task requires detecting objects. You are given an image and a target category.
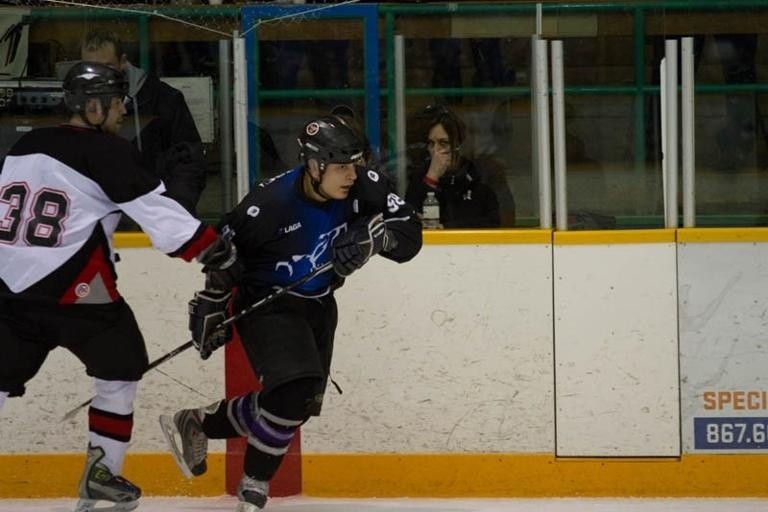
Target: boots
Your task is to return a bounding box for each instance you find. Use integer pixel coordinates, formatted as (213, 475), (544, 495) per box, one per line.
(173, 399), (221, 476)
(242, 467), (271, 508)
(77, 442), (142, 503)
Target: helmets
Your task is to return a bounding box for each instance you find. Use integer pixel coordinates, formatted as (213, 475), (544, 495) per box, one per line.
(297, 112), (368, 165)
(62, 60), (130, 111)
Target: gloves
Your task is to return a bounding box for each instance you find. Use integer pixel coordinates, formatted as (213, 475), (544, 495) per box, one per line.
(188, 290), (235, 359)
(200, 236), (248, 290)
(331, 211), (394, 279)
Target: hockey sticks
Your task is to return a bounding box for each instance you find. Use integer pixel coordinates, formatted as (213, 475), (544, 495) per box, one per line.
(58, 259), (337, 424)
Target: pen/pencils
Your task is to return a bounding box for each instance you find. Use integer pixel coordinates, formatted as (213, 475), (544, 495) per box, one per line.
(423, 146), (461, 162)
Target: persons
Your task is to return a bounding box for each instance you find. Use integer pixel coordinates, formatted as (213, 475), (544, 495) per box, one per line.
(169, 112), (419, 509)
(77, 26), (206, 225)
(400, 110), (498, 228)
(1, 60), (236, 503)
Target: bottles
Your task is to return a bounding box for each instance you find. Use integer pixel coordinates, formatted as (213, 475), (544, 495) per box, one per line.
(422, 191), (441, 232)
